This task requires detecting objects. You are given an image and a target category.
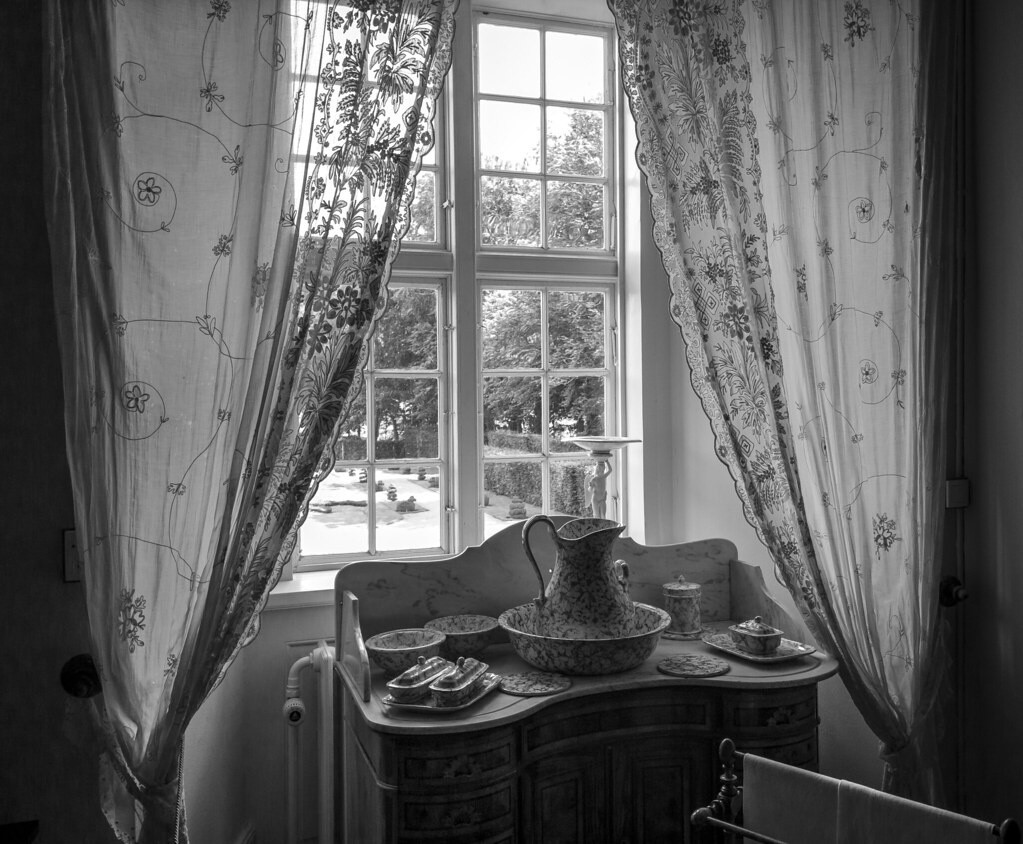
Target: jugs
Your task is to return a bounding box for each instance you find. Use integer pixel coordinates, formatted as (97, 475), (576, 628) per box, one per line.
(521, 513), (637, 640)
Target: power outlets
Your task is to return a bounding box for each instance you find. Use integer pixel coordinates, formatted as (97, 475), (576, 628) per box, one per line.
(64, 529), (84, 585)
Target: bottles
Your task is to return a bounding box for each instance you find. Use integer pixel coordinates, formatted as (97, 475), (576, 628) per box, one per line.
(662, 575), (704, 635)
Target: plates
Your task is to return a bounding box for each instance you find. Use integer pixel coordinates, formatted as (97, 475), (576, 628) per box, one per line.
(380, 672), (502, 713)
(700, 633), (818, 662)
(661, 623), (718, 641)
(498, 669), (572, 696)
(656, 652), (732, 677)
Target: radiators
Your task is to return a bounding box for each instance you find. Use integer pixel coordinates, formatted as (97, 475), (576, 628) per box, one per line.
(312, 644), (334, 844)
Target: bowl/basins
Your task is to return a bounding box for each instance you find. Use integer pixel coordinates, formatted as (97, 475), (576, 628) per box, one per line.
(364, 627), (446, 679)
(497, 600), (672, 673)
(727, 615), (785, 655)
(385, 655), (456, 705)
(428, 657), (489, 706)
(424, 614), (499, 662)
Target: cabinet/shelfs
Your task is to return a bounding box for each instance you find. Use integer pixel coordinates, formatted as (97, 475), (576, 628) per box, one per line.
(333, 513), (839, 844)
